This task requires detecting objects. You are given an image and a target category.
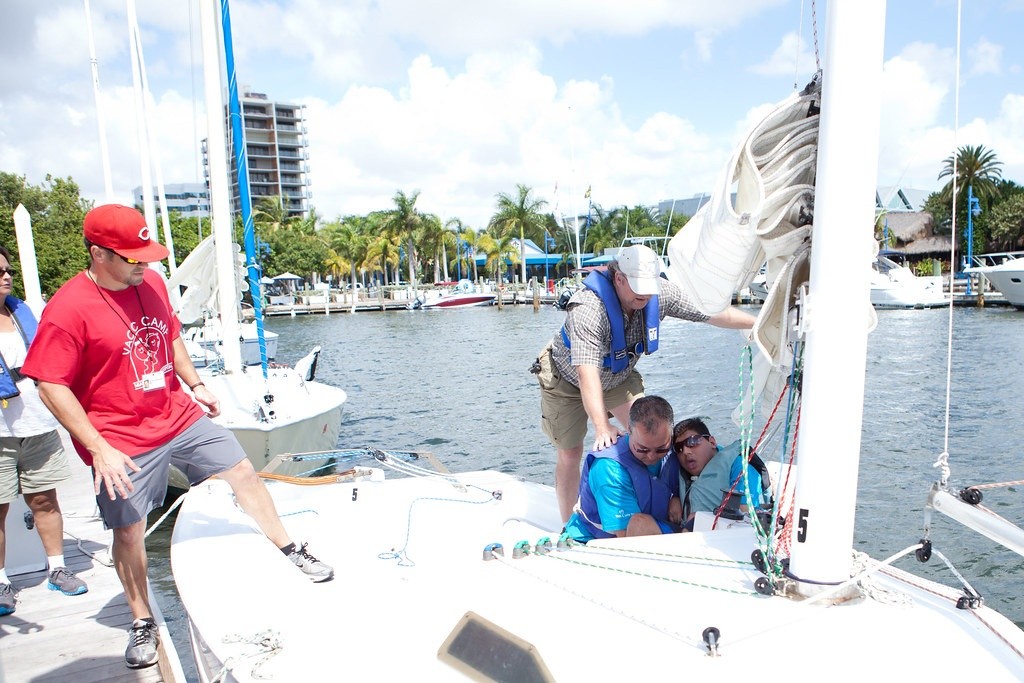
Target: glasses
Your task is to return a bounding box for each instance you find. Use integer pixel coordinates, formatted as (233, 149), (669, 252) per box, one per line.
(0, 269), (16, 277)
(673, 434), (708, 454)
(631, 431), (673, 453)
(97, 244), (143, 264)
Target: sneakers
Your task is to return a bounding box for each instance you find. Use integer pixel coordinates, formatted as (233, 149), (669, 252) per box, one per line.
(48, 567), (88, 596)
(287, 542), (333, 583)
(125, 616), (161, 668)
(0, 583), (19, 615)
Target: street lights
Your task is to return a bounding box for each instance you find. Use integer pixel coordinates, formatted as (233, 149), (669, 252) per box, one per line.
(964, 185), (984, 295)
(883, 218), (889, 273)
(544, 232), (557, 297)
(457, 234), (468, 281)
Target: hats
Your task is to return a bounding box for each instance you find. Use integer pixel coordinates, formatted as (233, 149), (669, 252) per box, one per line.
(83, 204), (169, 262)
(618, 244), (662, 295)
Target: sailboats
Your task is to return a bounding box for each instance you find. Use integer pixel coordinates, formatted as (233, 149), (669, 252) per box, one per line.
(0, 0), (1024, 683)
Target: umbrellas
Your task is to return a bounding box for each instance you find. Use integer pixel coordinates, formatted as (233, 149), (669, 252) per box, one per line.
(258, 271), (304, 285)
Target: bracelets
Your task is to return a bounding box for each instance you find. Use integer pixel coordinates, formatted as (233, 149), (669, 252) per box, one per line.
(190, 382), (205, 391)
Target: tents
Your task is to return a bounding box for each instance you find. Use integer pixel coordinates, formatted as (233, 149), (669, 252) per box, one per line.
(468, 251), (618, 273)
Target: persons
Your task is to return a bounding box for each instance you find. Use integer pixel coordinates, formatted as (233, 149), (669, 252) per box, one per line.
(626, 419), (774, 537)
(19, 205), (334, 667)
(0, 247), (87, 616)
(144, 380), (150, 388)
(559, 395), (681, 543)
(530, 244), (759, 536)
